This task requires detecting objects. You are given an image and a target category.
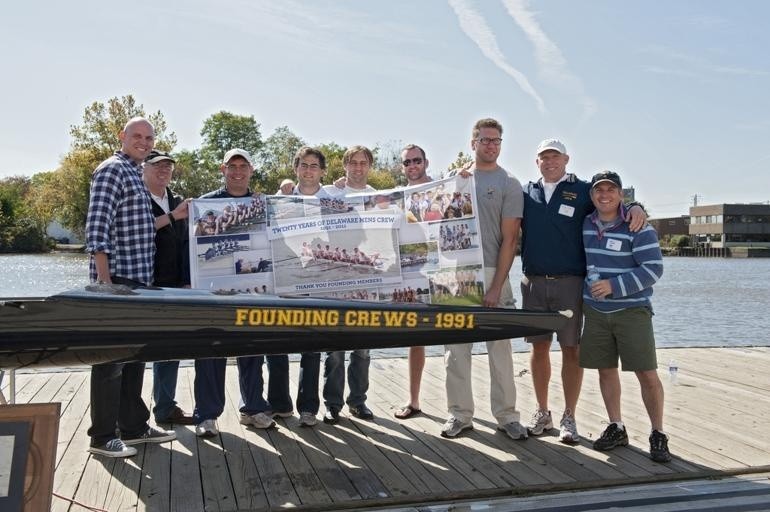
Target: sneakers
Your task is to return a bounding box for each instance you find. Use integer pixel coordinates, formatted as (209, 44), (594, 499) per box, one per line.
(648, 429), (672, 463)
(237, 410), (277, 430)
(119, 426), (178, 446)
(440, 415), (474, 438)
(87, 437), (138, 459)
(195, 416), (220, 437)
(496, 419), (529, 442)
(526, 409), (553, 437)
(558, 415), (580, 444)
(295, 411), (319, 427)
(593, 422), (629, 452)
(263, 406), (294, 419)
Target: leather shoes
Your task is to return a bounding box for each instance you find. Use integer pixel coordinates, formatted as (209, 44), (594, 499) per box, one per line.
(349, 403), (373, 420)
(321, 405), (340, 426)
(154, 407), (196, 426)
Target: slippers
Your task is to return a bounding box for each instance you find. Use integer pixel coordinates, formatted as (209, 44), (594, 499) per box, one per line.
(394, 404), (422, 420)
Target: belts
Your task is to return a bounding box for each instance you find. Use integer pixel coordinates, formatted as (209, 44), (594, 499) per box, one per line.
(531, 272), (569, 281)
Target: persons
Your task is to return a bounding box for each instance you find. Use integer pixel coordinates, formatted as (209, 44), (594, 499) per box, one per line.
(181, 147), (276, 437)
(300, 191), (484, 303)
(334, 144), (436, 420)
(85, 116), (194, 457)
(140, 149), (194, 425)
(200, 198), (271, 295)
(438, 117), (529, 440)
(578, 171), (674, 463)
(262, 146), (334, 427)
(280, 146), (377, 426)
(462, 139), (648, 442)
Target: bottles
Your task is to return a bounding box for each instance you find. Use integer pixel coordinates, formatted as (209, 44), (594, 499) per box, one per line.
(587, 265), (604, 302)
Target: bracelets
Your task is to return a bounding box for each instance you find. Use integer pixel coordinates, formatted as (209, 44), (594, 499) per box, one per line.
(168, 213), (176, 229)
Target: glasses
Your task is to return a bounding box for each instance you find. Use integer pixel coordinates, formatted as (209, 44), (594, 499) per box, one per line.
(400, 157), (424, 167)
(473, 137), (504, 146)
(224, 163), (250, 171)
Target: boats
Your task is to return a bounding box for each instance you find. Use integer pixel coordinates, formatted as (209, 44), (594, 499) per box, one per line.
(1, 278), (575, 372)
(204, 246), (249, 261)
(195, 216), (266, 236)
(299, 255), (390, 272)
(320, 203), (354, 212)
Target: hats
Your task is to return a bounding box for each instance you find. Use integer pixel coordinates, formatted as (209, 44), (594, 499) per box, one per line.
(590, 169), (623, 193)
(536, 138), (567, 157)
(223, 147), (252, 164)
(143, 147), (177, 165)
